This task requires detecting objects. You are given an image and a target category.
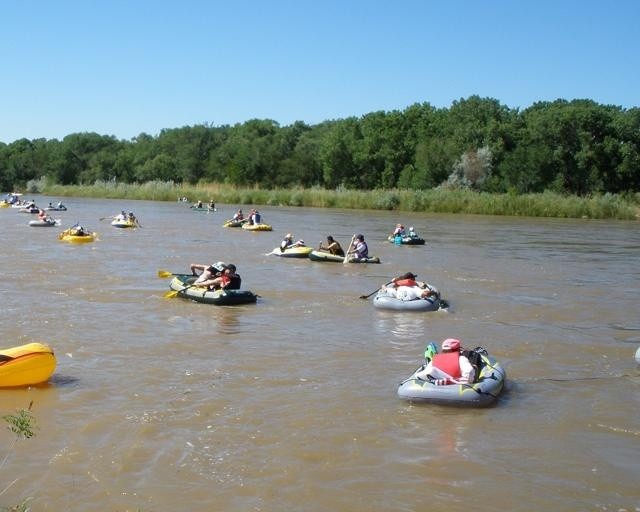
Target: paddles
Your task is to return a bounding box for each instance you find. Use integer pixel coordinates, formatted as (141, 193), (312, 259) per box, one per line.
(164, 286), (193, 299)
(360, 282), (392, 299)
(343, 234), (355, 265)
(99, 217), (116, 221)
(159, 271), (200, 277)
(424, 283), (449, 309)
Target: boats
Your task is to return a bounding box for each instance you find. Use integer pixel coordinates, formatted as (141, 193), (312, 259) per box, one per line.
(169, 274), (257, 307)
(0, 192), (274, 245)
(398, 348), (505, 407)
(309, 250), (380, 264)
(386, 232), (427, 246)
(272, 246), (315, 258)
(0, 342), (57, 390)
(635, 346), (640, 366)
(373, 279), (442, 312)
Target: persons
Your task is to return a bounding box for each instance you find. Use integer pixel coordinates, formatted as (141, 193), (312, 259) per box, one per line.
(233, 208), (261, 225)
(112, 210), (138, 225)
(392, 223), (417, 238)
(381, 271), (431, 301)
(317, 235), (344, 257)
(347, 233), (368, 259)
(207, 199), (214, 210)
(194, 200), (202, 208)
(182, 197), (187, 203)
(190, 261), (241, 291)
(3, 191), (87, 237)
(283, 233), (305, 248)
(425, 338), (478, 385)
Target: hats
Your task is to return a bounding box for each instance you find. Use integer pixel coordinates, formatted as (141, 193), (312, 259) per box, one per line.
(222, 264), (236, 272)
(442, 339), (460, 350)
(404, 273), (417, 279)
